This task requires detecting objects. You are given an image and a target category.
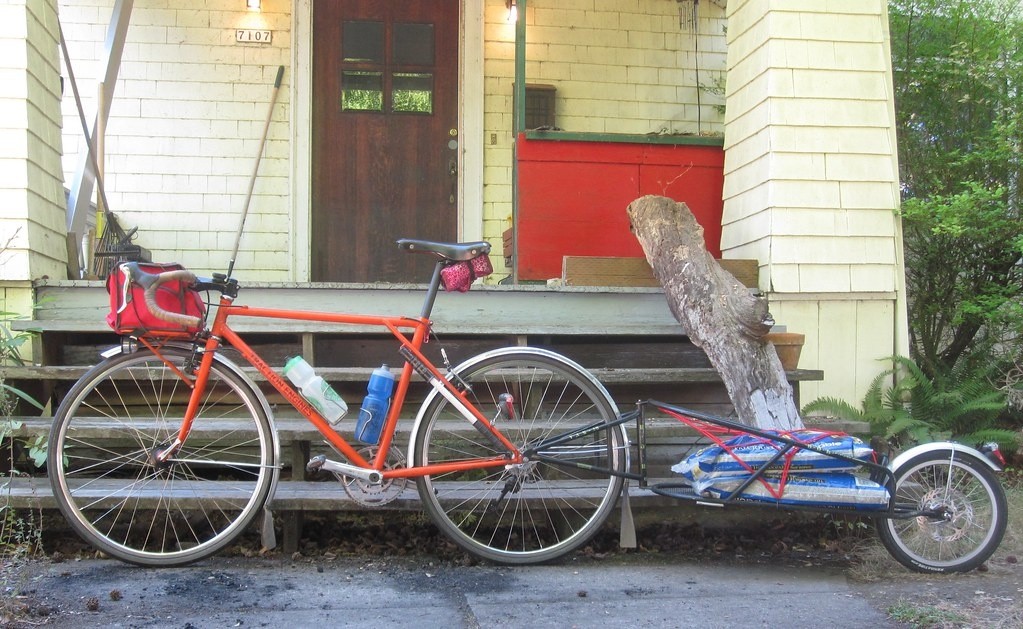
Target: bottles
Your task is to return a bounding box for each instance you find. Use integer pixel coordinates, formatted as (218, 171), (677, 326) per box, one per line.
(283, 355), (348, 427)
(353, 364), (395, 447)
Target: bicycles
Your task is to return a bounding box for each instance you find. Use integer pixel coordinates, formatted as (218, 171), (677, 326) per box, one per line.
(46, 237), (630, 567)
